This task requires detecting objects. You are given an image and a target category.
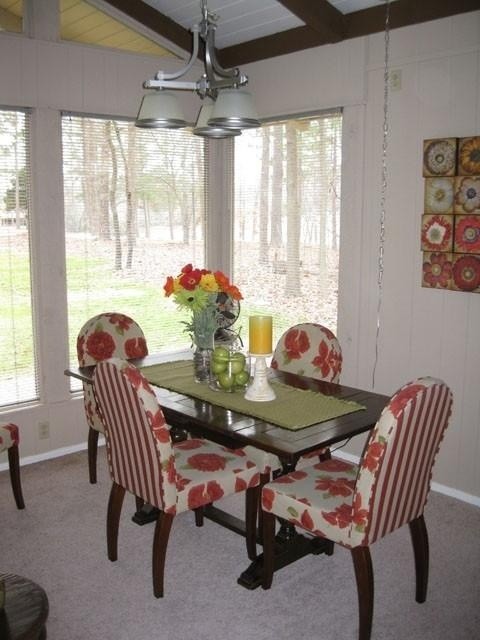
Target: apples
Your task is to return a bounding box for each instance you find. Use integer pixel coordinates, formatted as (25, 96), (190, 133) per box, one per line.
(211, 347), (250, 390)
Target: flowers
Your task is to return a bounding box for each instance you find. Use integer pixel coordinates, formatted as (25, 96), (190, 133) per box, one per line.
(163, 263), (243, 342)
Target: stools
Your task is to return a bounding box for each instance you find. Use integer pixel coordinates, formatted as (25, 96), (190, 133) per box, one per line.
(0, 572), (47, 638)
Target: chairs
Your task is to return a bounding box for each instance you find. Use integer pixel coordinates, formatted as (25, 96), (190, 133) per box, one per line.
(78, 313), (150, 482)
(261, 374), (456, 640)
(91, 357), (263, 598)
(271, 320), (343, 387)
(0, 416), (26, 512)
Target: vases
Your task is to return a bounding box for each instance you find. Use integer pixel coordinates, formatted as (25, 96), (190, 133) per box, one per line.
(192, 335), (214, 384)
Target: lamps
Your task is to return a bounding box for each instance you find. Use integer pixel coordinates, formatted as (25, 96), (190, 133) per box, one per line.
(136, 7), (262, 140)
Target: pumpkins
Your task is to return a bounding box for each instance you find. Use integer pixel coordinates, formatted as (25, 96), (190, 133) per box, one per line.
(461, 136), (480, 174)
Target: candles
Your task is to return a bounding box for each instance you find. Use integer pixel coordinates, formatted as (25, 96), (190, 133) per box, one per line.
(249, 316), (272, 355)
